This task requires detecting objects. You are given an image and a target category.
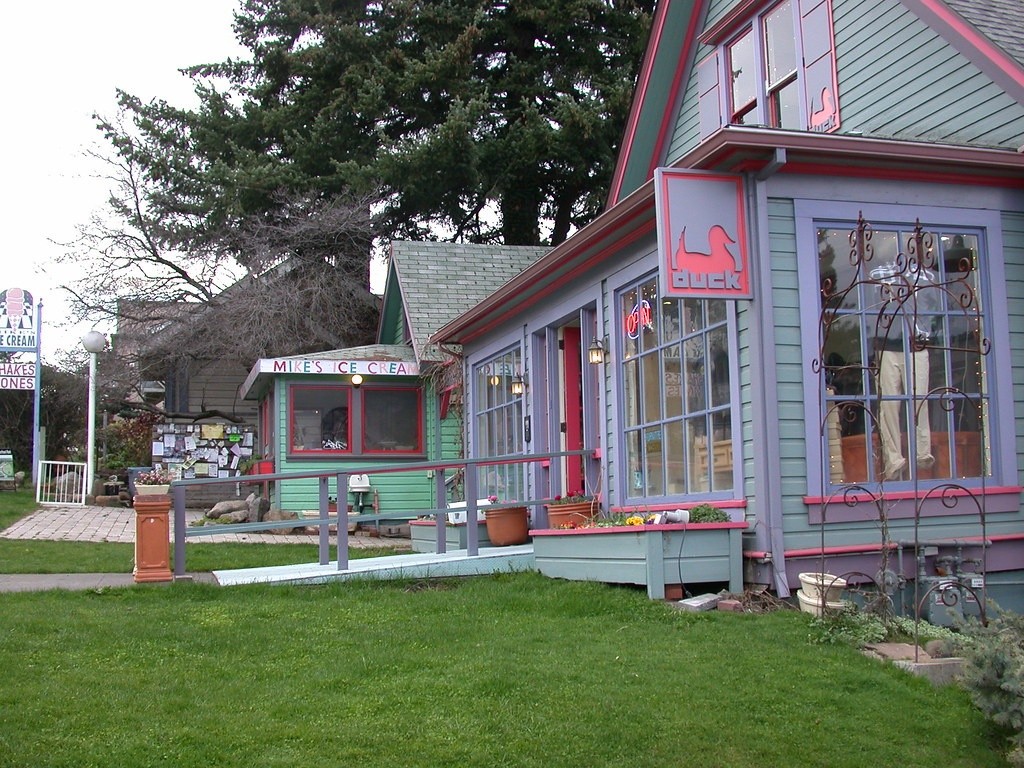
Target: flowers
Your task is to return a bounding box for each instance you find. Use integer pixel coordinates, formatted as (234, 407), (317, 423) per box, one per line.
(134, 468), (174, 485)
(550, 491), (596, 505)
(480, 495), (531, 522)
(556, 503), (733, 529)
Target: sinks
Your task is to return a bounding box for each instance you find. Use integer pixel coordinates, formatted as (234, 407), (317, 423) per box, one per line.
(349, 484), (370, 492)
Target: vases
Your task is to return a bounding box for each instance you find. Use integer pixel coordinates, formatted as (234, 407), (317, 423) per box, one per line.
(303, 510), (360, 531)
(799, 572), (848, 601)
(529, 521), (748, 601)
(132, 482), (170, 495)
(485, 507), (529, 546)
(42, 485), (53, 493)
(547, 501), (598, 528)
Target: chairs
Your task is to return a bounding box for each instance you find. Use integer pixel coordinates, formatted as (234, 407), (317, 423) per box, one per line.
(0, 455), (17, 492)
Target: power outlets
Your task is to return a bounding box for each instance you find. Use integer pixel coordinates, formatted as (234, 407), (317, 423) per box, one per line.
(428, 470), (432, 478)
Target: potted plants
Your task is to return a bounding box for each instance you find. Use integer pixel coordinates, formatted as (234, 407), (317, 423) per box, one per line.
(408, 515), (492, 553)
(238, 454), (275, 487)
(395, 442), (414, 450)
(378, 432), (396, 450)
(329, 496), (353, 512)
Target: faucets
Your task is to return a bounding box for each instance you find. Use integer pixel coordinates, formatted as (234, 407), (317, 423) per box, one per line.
(357, 477), (363, 481)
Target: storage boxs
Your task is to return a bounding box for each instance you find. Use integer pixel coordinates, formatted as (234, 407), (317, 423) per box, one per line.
(447, 500), (493, 524)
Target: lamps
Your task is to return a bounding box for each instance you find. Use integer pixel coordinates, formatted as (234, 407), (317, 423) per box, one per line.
(661, 510), (691, 524)
(511, 369), (530, 394)
(588, 334), (611, 364)
(352, 375), (363, 389)
